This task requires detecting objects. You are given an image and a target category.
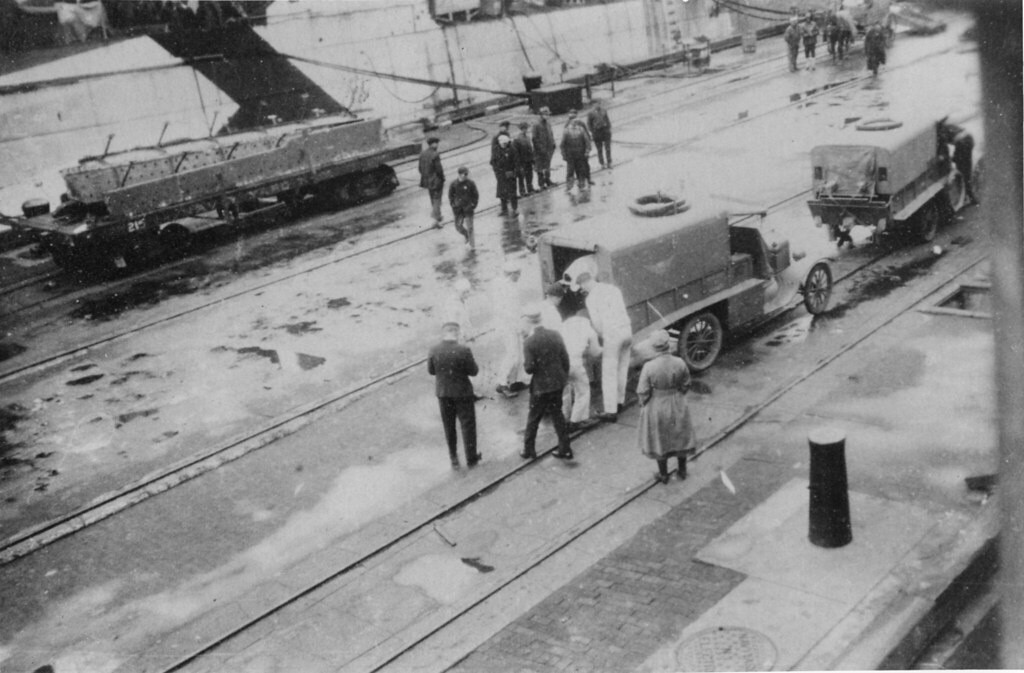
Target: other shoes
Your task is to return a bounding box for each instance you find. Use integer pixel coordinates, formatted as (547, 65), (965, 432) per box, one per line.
(617, 403), (624, 412)
(676, 468), (687, 479)
(598, 412), (618, 423)
(565, 420), (591, 433)
(468, 452), (482, 467)
(654, 471), (669, 484)
(519, 449), (537, 459)
(552, 450), (574, 459)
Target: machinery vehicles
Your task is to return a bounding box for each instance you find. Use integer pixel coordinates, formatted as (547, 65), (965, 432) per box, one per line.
(1, 0), (427, 280)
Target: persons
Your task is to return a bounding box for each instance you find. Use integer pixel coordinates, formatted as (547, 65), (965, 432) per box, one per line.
(638, 330), (697, 484)
(448, 167), (479, 251)
(449, 257), (632, 430)
(426, 321), (481, 469)
(490, 98), (613, 220)
(419, 137), (446, 229)
(785, 10), (887, 79)
(518, 305), (574, 460)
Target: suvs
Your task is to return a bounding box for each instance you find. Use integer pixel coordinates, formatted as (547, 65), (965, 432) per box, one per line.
(805, 110), (970, 242)
(538, 189), (832, 371)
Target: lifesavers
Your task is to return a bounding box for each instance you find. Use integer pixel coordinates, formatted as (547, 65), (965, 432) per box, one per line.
(855, 115), (902, 131)
(627, 188), (687, 217)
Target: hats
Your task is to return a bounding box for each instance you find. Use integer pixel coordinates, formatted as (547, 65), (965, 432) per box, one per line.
(538, 106), (553, 116)
(457, 167), (474, 175)
(651, 328), (669, 350)
